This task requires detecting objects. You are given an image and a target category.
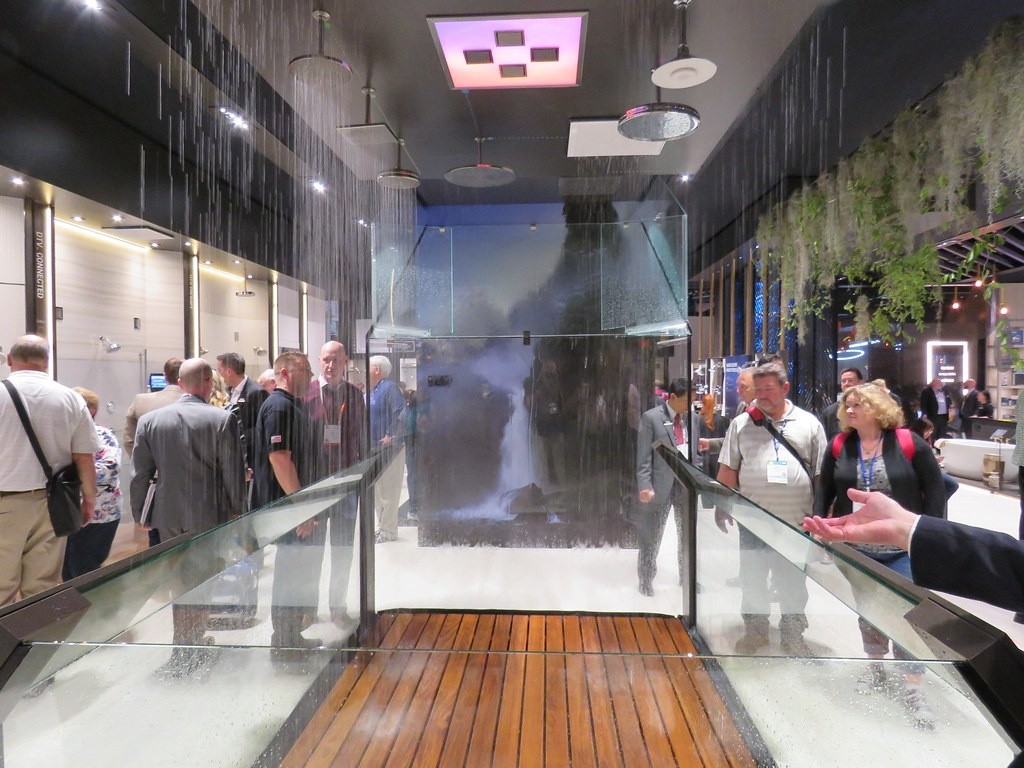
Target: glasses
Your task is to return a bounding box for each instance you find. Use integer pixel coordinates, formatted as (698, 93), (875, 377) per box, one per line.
(924, 430), (933, 436)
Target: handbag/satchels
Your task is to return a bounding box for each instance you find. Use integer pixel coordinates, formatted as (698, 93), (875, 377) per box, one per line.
(46, 465), (82, 537)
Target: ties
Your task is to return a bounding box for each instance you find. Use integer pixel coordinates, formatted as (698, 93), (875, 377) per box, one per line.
(673, 414), (683, 445)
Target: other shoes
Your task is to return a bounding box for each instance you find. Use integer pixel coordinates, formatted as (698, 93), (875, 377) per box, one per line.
(301, 611), (315, 632)
(681, 578), (702, 595)
(329, 608), (352, 630)
(374, 530), (397, 542)
(173, 632), (219, 680)
(1014, 613), (1024, 623)
(294, 636), (324, 654)
(855, 664), (886, 694)
(639, 578), (654, 598)
(270, 648), (313, 677)
(901, 685), (936, 731)
(22, 678), (54, 699)
(406, 511), (417, 520)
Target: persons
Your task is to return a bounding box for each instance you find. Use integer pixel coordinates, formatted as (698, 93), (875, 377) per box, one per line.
(61, 388), (123, 582)
(369, 355), (411, 543)
(800, 489), (1023, 619)
(957, 377), (994, 440)
(697, 353), (961, 733)
(1, 334), (102, 612)
(123, 341), (367, 680)
(634, 376), (706, 596)
(1010, 390), (1024, 625)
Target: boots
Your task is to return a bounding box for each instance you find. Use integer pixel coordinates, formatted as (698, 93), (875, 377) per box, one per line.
(735, 616), (771, 655)
(778, 621), (823, 667)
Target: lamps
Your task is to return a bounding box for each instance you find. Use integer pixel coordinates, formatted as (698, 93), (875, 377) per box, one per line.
(425, 9), (588, 91)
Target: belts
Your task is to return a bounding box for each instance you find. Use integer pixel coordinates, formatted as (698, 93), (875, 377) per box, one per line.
(0, 488), (45, 497)
